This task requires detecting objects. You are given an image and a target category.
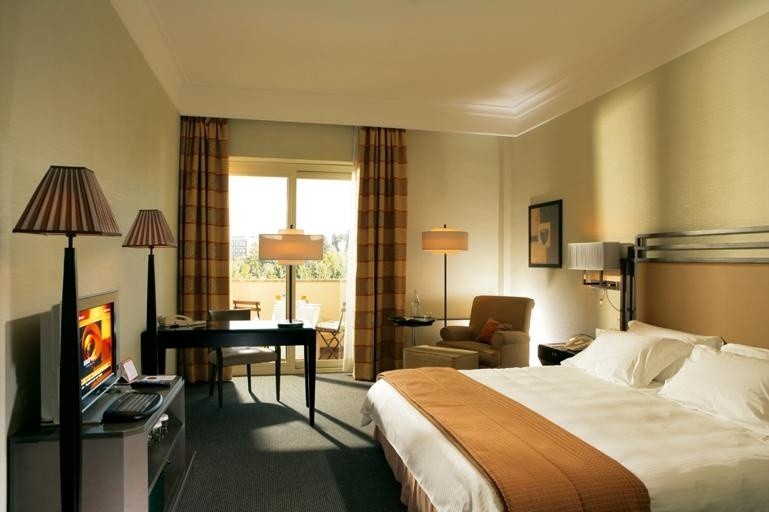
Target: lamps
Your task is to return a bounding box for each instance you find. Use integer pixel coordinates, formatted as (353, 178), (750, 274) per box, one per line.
(258, 233), (324, 328)
(566, 241), (622, 291)
(13, 165), (123, 512)
(122, 209), (179, 375)
(422, 224), (469, 327)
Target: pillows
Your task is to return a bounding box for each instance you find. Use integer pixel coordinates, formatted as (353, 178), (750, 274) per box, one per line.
(658, 345), (769, 438)
(626, 320), (725, 383)
(560, 329), (693, 389)
(477, 320), (500, 345)
(718, 341), (769, 359)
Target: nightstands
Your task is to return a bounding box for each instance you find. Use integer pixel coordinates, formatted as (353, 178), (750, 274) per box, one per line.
(537, 342), (583, 365)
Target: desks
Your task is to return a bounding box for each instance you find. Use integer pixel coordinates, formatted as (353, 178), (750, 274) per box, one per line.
(156, 320), (317, 428)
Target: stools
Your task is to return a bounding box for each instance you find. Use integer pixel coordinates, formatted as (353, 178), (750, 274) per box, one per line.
(404, 344), (479, 370)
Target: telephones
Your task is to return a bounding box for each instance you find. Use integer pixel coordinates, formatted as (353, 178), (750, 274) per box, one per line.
(552, 333), (594, 352)
(158, 314), (192, 326)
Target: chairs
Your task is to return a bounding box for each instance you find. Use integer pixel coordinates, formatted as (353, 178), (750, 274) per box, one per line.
(234, 300), (270, 349)
(208, 308), (282, 409)
(317, 303), (346, 360)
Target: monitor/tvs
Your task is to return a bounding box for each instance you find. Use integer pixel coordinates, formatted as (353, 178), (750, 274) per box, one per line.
(36, 290), (121, 425)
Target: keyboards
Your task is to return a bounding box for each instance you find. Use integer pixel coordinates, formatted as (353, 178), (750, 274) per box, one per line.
(102, 391), (164, 424)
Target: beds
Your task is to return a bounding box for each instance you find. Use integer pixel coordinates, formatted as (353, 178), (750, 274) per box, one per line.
(361, 226), (769, 511)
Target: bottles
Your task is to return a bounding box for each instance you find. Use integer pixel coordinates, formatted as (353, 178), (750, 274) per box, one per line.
(410, 289), (420, 317)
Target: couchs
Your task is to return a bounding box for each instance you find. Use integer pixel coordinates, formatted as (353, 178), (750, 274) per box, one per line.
(437, 295), (535, 367)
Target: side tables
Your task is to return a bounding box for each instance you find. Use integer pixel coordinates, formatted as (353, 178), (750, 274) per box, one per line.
(387, 315), (435, 347)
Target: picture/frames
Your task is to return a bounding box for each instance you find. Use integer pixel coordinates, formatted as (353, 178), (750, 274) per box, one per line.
(527, 199), (564, 268)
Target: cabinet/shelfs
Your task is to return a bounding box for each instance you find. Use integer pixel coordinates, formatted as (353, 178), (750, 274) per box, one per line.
(7, 375), (197, 512)
